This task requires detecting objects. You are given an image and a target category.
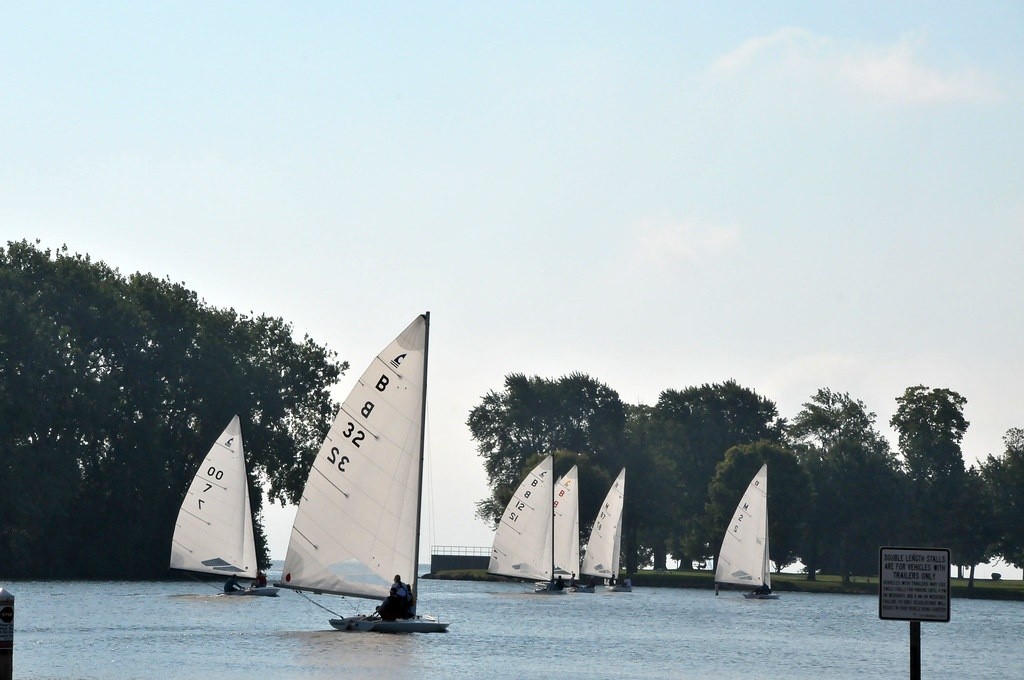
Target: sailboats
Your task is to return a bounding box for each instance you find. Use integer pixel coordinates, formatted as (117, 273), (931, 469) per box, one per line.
(585, 465), (632, 591)
(714, 462), (780, 599)
(169, 413), (281, 597)
(280, 311), (449, 634)
(487, 454), (594, 594)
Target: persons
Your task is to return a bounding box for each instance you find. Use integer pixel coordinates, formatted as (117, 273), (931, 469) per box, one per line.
(552, 575), (565, 591)
(569, 573), (579, 588)
(223, 573), (245, 593)
(622, 577), (632, 587)
(609, 575), (616, 586)
(587, 578), (596, 588)
(377, 587), (403, 621)
(392, 575), (409, 599)
(752, 584), (772, 596)
(250, 571), (266, 588)
(547, 580), (556, 589)
